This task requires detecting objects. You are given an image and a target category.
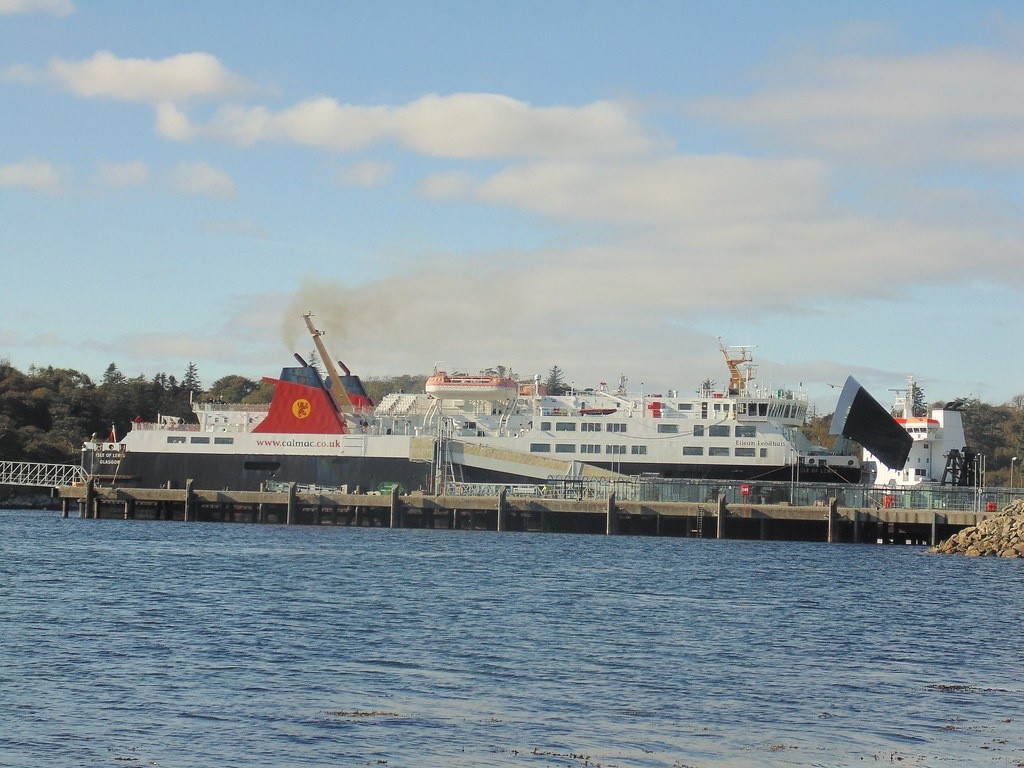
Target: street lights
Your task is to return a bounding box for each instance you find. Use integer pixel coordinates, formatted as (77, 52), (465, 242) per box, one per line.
(1010, 456), (1018, 504)
(977, 452), (983, 513)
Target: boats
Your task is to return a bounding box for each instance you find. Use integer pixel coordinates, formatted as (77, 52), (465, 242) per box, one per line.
(76, 422), (661, 505)
(184, 309), (880, 505)
(862, 374), (970, 510)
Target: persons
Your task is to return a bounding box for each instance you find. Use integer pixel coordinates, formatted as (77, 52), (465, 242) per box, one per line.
(105, 444), (118, 452)
(135, 415), (142, 430)
(178, 417), (184, 424)
(162, 417), (167, 425)
